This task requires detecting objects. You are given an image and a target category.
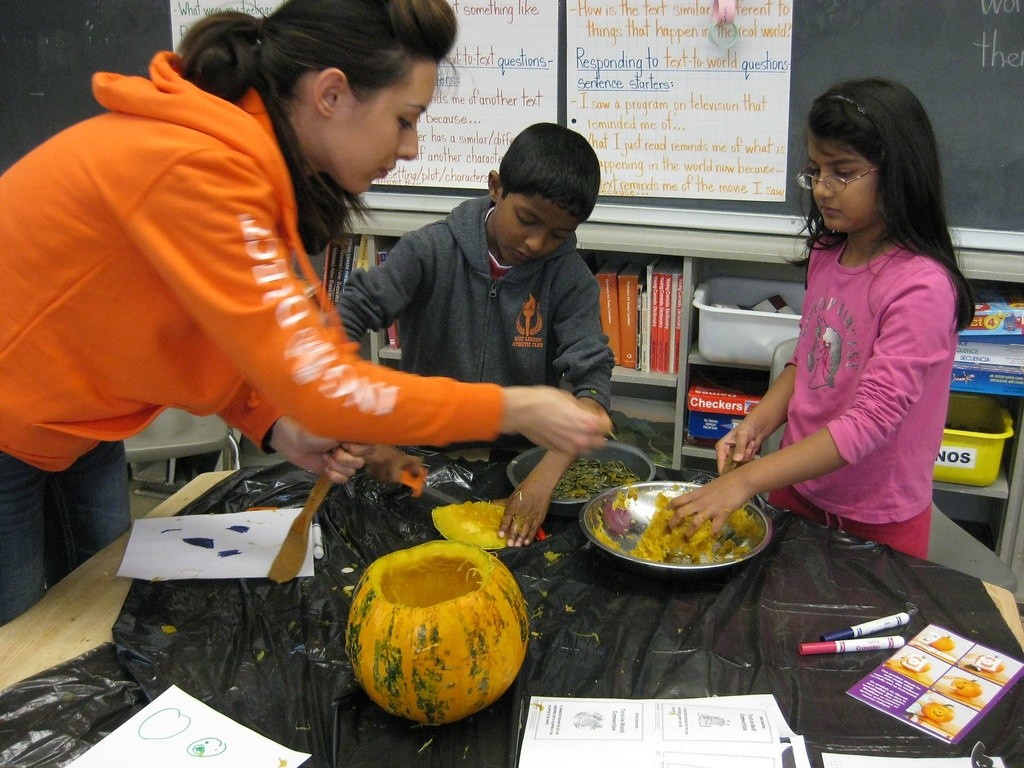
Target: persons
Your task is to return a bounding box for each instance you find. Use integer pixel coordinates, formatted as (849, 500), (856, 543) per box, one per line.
(0, 0), (612, 631)
(662, 76), (976, 563)
(334, 122), (616, 548)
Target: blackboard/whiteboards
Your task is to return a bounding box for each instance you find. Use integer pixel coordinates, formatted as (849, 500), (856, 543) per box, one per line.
(0, 0), (1024, 251)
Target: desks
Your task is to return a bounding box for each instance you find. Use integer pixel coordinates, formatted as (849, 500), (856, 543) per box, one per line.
(0, 467), (1024, 768)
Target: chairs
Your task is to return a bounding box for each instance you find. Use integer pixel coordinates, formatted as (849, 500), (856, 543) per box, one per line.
(759, 335), (1020, 597)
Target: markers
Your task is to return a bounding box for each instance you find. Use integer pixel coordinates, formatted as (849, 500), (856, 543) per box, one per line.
(821, 612), (910, 642)
(798, 635), (906, 655)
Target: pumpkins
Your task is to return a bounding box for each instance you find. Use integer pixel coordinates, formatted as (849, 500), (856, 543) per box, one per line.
(346, 539), (532, 725)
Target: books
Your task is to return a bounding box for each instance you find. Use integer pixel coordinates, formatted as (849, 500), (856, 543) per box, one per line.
(319, 232), (686, 373)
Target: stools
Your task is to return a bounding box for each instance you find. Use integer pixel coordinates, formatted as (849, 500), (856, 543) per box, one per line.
(122, 406), (243, 489)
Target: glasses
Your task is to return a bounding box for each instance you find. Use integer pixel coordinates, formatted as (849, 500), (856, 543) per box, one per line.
(796, 164), (881, 191)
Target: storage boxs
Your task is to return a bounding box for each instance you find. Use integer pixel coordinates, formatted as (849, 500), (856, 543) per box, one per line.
(951, 275), (1024, 399)
(690, 271), (809, 368)
(686, 366), (770, 453)
(929, 388), (1014, 488)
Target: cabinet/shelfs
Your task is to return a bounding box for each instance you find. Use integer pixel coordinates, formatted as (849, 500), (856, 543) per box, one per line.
(307, 211), (1024, 570)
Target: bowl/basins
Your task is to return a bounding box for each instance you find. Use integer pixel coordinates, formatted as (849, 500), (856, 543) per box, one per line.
(581, 481), (777, 576)
(507, 439), (656, 515)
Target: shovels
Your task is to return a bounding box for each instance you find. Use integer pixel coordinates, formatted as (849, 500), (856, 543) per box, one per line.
(265, 471), (334, 584)
